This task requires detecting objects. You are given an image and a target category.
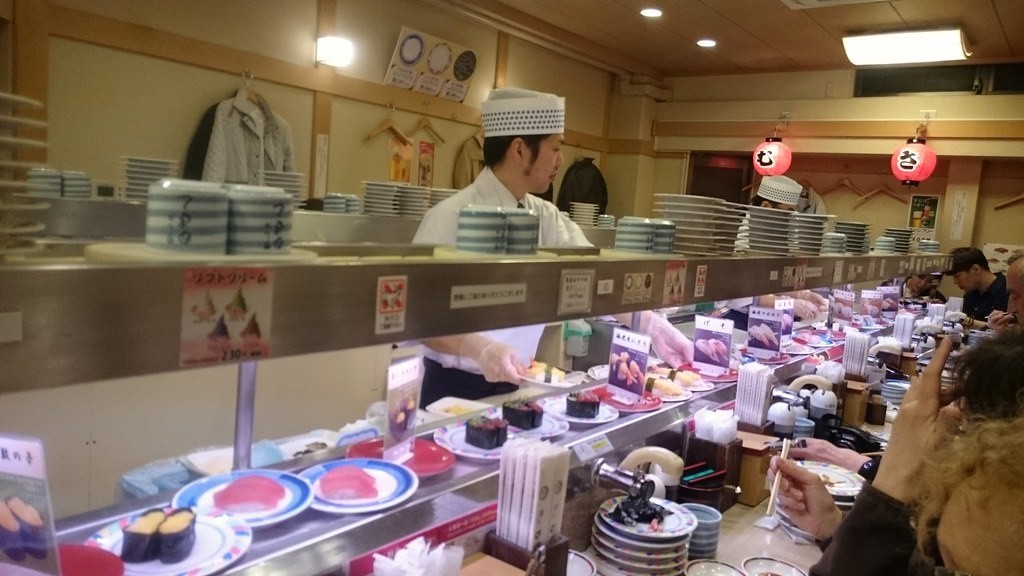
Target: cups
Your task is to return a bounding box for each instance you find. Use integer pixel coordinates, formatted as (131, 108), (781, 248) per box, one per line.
(926, 241), (939, 253)
(874, 237), (895, 255)
(224, 183), (292, 255)
(456, 205), (507, 255)
(880, 385), (906, 408)
(145, 178), (228, 255)
(614, 216), (654, 253)
(324, 192), (359, 216)
(61, 171), (91, 200)
(598, 214), (615, 228)
(793, 417), (815, 437)
(680, 503), (722, 559)
(677, 482), (724, 512)
(822, 233), (843, 254)
(650, 219), (675, 253)
(24, 167), (61, 199)
(918, 240), (928, 252)
(502, 208), (539, 255)
(842, 234), (847, 254)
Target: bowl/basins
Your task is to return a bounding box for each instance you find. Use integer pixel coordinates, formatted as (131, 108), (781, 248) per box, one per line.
(425, 397), (490, 416)
(742, 556), (807, 576)
(684, 560), (746, 576)
(566, 549), (597, 576)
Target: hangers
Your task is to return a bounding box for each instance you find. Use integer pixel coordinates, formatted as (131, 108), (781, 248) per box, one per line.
(242, 69), (262, 106)
(797, 178), (815, 190)
(364, 101), (414, 148)
(740, 176), (762, 193)
(855, 174), (908, 204)
(994, 191), (1024, 208)
(817, 164), (868, 199)
(406, 98), (446, 143)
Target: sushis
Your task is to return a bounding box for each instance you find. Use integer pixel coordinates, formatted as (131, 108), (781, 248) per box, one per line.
(862, 303), (880, 318)
(389, 398), (415, 441)
(502, 398), (544, 431)
(567, 392), (600, 419)
(121, 508), (196, 564)
(748, 323), (779, 346)
(882, 297), (897, 310)
(833, 302), (852, 317)
(782, 313), (793, 330)
(0, 496), (47, 563)
(696, 338), (728, 362)
(213, 465), (380, 510)
(611, 351), (645, 386)
(646, 368), (703, 395)
(463, 415), (507, 450)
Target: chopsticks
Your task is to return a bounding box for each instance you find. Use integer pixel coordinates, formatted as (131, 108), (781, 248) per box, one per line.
(766, 437), (790, 517)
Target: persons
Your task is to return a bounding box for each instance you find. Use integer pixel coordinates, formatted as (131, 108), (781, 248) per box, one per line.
(880, 272), (947, 304)
(394, 87), (695, 410)
(769, 249), (1024, 576)
(945, 247), (1008, 330)
(715, 174), (826, 331)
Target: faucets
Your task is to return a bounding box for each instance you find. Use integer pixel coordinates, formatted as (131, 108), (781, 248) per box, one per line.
(943, 321), (954, 329)
(771, 386), (810, 412)
(867, 355), (883, 369)
(912, 334), (928, 344)
(591, 459), (655, 501)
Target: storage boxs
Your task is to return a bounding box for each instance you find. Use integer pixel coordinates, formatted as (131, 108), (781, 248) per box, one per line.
(962, 335), (970, 345)
(686, 435), (744, 515)
(843, 380), (871, 428)
(921, 333), (938, 343)
(457, 552), (526, 576)
(865, 401), (888, 426)
(900, 352), (919, 377)
(951, 342), (960, 352)
(483, 530), (570, 576)
(833, 382), (848, 427)
(844, 373), (869, 395)
(902, 347), (914, 353)
(737, 419), (775, 437)
(736, 430), (780, 507)
(877, 351), (903, 370)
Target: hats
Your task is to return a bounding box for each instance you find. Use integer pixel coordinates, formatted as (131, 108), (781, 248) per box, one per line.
(481, 88), (566, 137)
(757, 174), (802, 205)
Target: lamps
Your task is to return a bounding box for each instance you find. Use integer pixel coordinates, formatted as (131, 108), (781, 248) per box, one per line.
(316, 35), (355, 69)
(841, 23), (977, 67)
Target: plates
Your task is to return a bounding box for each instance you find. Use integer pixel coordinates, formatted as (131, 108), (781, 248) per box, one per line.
(171, 470), (315, 532)
(535, 396), (619, 424)
(584, 300), (895, 413)
(793, 462), (865, 509)
(261, 171), (307, 214)
(517, 374), (571, 388)
(569, 202), (599, 229)
(119, 155), (179, 202)
(360, 181), (461, 221)
(348, 440), (455, 477)
(481, 406), (569, 438)
(298, 458), (418, 515)
(653, 194), (914, 258)
(84, 507), (252, 576)
(591, 494), (698, 576)
(434, 420), (540, 461)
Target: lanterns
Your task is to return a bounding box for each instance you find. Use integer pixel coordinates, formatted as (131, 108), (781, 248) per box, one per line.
(753, 137), (792, 176)
(890, 138), (937, 186)
